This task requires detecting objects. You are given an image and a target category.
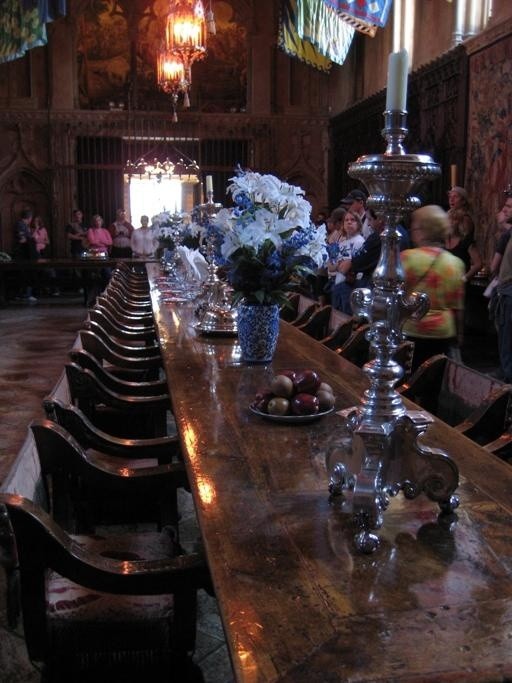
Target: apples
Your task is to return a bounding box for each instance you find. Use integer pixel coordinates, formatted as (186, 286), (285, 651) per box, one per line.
(253, 388), (273, 402)
(282, 371), (297, 379)
(293, 369), (321, 393)
(291, 393), (320, 415)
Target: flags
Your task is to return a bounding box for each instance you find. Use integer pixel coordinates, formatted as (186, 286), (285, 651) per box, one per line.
(0, 0), (66, 64)
(296, 0), (356, 66)
(324, 1), (393, 28)
(325, 4), (379, 39)
(275, 0), (333, 76)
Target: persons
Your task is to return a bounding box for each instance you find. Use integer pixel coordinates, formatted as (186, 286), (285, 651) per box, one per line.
(314, 187), (512, 408)
(87, 215), (112, 281)
(66, 210), (88, 294)
(131, 216), (155, 259)
(108, 209), (134, 258)
(31, 215), (50, 256)
(13, 210), (37, 301)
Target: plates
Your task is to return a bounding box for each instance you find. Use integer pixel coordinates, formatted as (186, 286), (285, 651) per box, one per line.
(151, 275), (188, 303)
(192, 322), (238, 336)
(248, 400), (335, 424)
(78, 258), (109, 261)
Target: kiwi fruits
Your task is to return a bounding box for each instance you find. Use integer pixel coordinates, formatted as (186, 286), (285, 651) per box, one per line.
(315, 391), (334, 410)
(268, 398), (291, 414)
(319, 382), (333, 394)
(269, 377), (293, 397)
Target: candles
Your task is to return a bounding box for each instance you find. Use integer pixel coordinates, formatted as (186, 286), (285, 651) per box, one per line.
(387, 50), (408, 111)
(207, 175), (212, 193)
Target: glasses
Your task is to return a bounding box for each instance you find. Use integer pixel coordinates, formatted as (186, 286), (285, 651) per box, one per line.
(408, 227), (421, 232)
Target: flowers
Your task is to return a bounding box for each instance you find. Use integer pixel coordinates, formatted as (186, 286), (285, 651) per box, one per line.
(150, 212), (203, 250)
(206, 163), (366, 311)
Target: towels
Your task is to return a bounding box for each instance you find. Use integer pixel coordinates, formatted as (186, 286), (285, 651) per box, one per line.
(177, 245), (207, 283)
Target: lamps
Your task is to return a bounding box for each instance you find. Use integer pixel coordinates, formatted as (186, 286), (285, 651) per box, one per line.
(163, 0), (216, 108)
(157, 46), (188, 123)
(121, 120), (201, 185)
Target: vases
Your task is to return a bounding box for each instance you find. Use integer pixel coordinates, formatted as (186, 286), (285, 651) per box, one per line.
(237, 302), (280, 365)
(164, 248), (174, 263)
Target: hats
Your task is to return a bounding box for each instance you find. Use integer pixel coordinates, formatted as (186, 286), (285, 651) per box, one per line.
(326, 208), (347, 225)
(340, 189), (366, 205)
(448, 186), (469, 200)
